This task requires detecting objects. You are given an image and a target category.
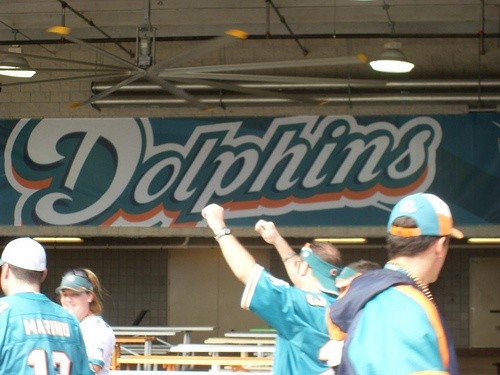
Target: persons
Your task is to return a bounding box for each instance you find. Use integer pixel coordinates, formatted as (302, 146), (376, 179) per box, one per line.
(0, 237), (93, 375)
(56, 267), (117, 375)
(330, 192), (465, 375)
(200, 203), (383, 375)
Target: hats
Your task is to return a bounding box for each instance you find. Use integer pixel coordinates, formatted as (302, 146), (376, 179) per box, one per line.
(300, 247), (343, 296)
(335, 265), (363, 288)
(387, 191), (464, 239)
(0, 237), (46, 272)
(56, 274), (94, 292)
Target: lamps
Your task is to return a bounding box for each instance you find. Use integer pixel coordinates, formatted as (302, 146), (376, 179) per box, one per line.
(369, 21), (418, 73)
(0, 30), (36, 78)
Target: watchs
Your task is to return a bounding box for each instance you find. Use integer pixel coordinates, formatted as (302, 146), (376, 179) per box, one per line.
(214, 227), (232, 241)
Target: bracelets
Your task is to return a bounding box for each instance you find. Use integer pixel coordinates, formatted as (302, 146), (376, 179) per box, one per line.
(283, 252), (296, 263)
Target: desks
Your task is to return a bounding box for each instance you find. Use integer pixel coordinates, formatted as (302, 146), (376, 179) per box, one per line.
(109, 327), (277, 375)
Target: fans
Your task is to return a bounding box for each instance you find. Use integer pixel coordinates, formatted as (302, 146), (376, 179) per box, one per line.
(0, 0), (388, 113)
